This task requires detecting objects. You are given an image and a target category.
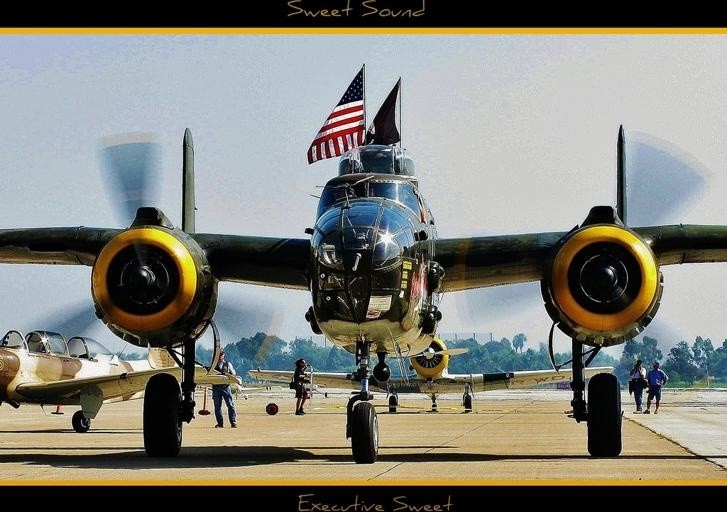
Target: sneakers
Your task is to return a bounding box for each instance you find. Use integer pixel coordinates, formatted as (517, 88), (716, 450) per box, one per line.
(633, 410), (659, 414)
(295, 407), (306, 415)
(214, 419), (223, 428)
(230, 424), (238, 428)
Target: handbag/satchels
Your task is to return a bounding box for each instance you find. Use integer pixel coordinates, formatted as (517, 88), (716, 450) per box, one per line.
(639, 378), (648, 389)
(289, 381), (301, 389)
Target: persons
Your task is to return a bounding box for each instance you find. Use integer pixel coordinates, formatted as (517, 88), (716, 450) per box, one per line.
(294, 359), (312, 415)
(628, 359), (648, 415)
(642, 362), (670, 415)
(207, 352), (240, 429)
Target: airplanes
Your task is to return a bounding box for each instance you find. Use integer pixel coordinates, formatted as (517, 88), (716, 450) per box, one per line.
(0, 125), (727, 465)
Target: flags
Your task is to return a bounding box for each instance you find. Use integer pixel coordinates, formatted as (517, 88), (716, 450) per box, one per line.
(306, 62), (366, 168)
(360, 77), (401, 148)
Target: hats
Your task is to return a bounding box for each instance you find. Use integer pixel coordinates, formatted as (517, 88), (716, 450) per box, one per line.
(220, 348), (226, 356)
(652, 361), (660, 365)
